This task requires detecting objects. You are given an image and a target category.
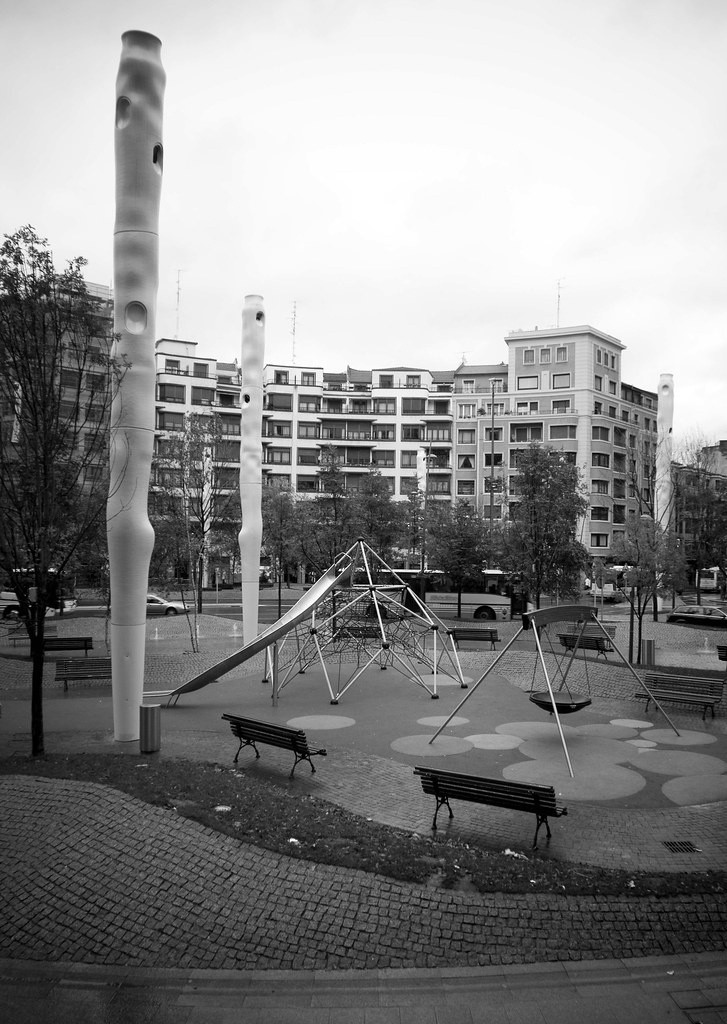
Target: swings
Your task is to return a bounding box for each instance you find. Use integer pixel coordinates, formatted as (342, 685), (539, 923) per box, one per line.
(528, 618), (592, 714)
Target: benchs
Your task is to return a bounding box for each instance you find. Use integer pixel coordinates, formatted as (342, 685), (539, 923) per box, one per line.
(55, 656), (111, 691)
(43, 636), (93, 656)
(334, 626), (383, 642)
(447, 627), (501, 650)
(221, 712), (327, 780)
(7, 625), (58, 647)
(556, 624), (617, 660)
(412, 765), (568, 852)
(716, 645), (727, 661)
(635, 673), (724, 720)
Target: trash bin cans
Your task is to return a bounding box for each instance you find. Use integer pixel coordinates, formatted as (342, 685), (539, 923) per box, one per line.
(139, 703), (163, 752)
(641, 637), (656, 666)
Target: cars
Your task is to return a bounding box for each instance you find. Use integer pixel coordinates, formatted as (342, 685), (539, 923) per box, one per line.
(147, 593), (191, 616)
(261, 556), (274, 585)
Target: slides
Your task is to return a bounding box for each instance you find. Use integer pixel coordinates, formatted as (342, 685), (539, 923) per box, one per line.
(142, 561), (351, 699)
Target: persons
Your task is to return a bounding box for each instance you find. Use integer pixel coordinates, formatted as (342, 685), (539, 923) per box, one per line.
(490, 584), (514, 597)
(27, 582), (37, 620)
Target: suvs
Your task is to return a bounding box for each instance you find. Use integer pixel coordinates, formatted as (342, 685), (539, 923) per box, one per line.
(667, 605), (727, 628)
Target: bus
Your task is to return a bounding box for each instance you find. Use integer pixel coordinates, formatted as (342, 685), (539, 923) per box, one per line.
(696, 566), (727, 593)
(350, 567), (511, 622)
(0, 568), (76, 601)
(590, 565), (651, 602)
